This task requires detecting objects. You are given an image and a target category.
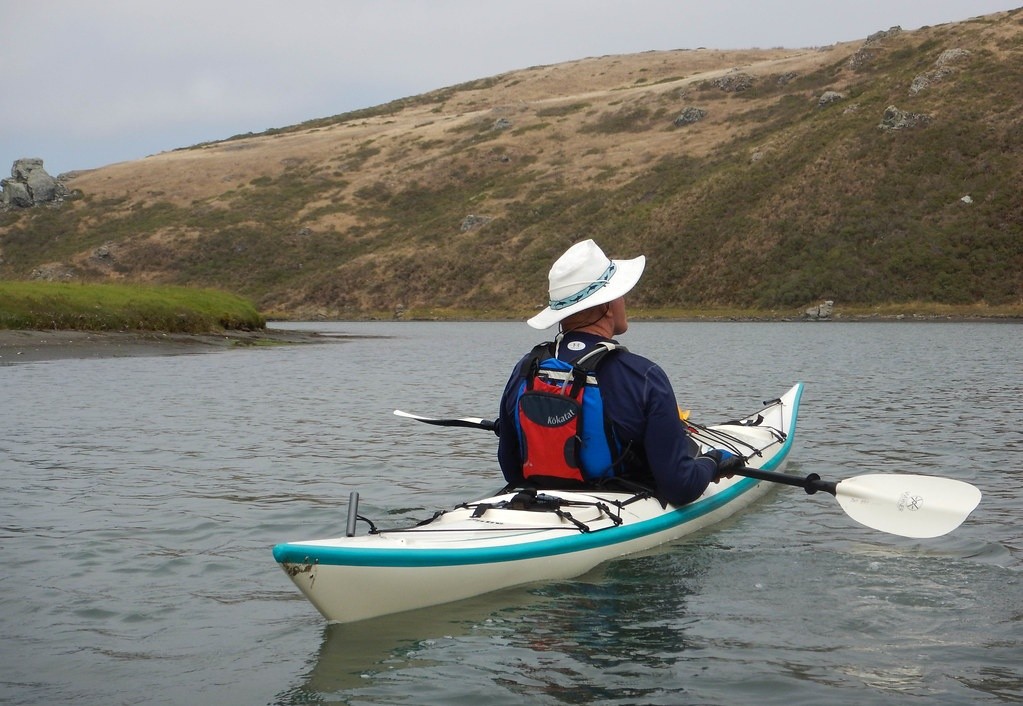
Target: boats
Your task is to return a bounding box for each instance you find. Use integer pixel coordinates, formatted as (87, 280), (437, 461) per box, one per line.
(271, 381), (806, 628)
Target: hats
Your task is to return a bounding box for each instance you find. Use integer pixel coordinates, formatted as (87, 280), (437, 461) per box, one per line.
(527, 239), (646, 329)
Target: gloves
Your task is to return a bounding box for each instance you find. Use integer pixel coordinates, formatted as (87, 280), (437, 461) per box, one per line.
(696, 449), (745, 484)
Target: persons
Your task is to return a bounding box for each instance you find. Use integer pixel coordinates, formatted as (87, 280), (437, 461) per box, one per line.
(498, 239), (745, 506)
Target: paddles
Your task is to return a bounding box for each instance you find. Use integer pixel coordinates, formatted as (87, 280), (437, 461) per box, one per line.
(393, 409), (982, 539)
